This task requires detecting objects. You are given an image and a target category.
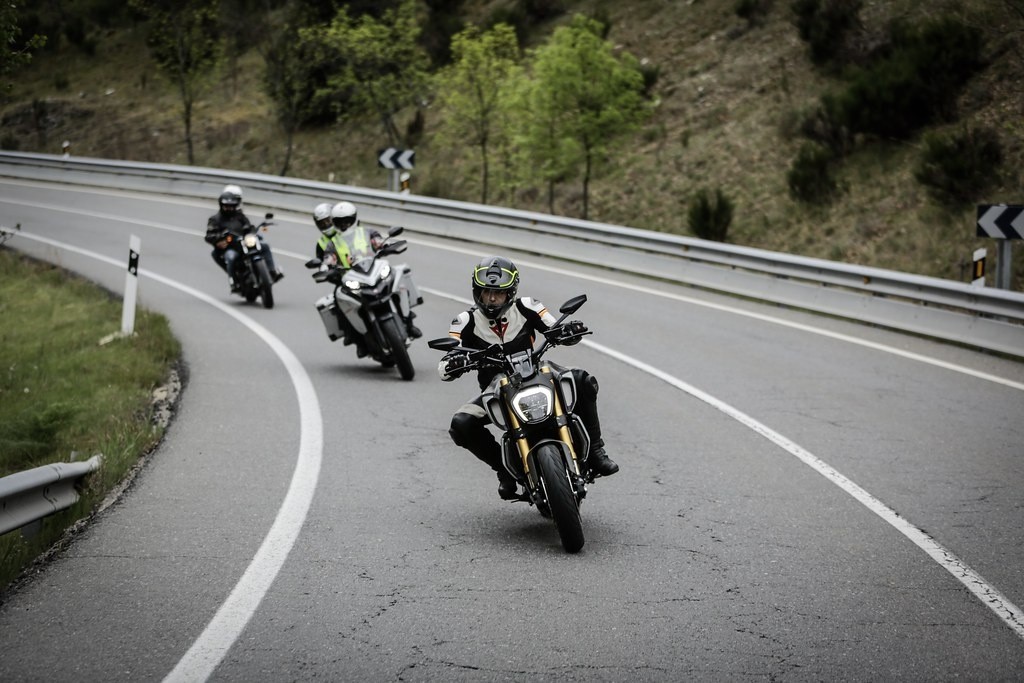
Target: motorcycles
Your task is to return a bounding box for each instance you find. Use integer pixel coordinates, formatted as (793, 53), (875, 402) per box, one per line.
(221, 214), (285, 310)
(305, 227), (417, 380)
(428, 293), (604, 554)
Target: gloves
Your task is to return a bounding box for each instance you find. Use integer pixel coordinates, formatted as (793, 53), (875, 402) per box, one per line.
(556, 319), (585, 346)
(446, 355), (470, 375)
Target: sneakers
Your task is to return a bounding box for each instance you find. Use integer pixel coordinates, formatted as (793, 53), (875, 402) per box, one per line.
(498, 472), (518, 497)
(586, 441), (620, 478)
(402, 317), (424, 339)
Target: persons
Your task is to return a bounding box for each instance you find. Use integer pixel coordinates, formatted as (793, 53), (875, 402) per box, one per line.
(205, 191), (285, 290)
(312, 204), (415, 344)
(212, 184), (275, 289)
(438, 258), (621, 496)
(319, 201), (423, 359)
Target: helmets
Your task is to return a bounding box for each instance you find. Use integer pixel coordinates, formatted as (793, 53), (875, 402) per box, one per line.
(221, 186), (243, 198)
(471, 256), (520, 319)
(332, 201), (360, 233)
(217, 193), (240, 206)
(312, 202), (336, 237)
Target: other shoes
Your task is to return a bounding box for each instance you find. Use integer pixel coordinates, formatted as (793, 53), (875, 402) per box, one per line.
(354, 340), (372, 359)
(270, 269), (283, 283)
